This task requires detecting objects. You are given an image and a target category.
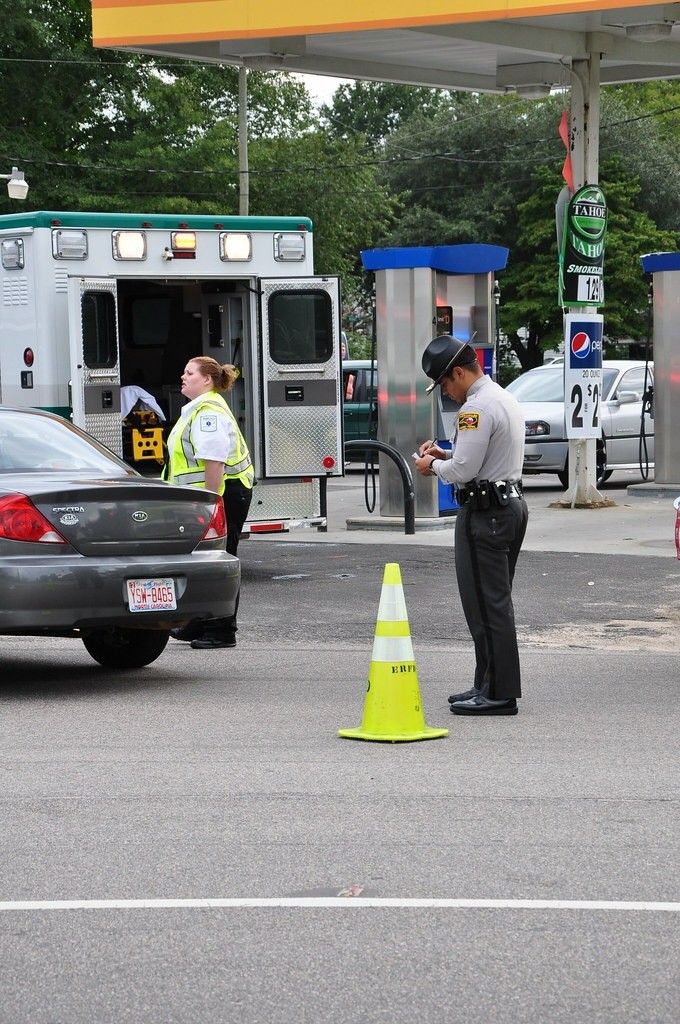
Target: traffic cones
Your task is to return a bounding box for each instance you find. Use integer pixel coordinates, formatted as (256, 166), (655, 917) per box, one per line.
(337, 562), (450, 742)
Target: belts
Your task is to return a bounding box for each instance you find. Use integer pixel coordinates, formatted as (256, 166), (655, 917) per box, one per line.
(501, 482), (523, 500)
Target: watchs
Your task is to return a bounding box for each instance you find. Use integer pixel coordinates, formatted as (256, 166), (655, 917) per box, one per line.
(428, 459), (438, 476)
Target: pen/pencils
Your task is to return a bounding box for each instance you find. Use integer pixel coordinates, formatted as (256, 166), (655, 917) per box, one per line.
(420, 437), (438, 458)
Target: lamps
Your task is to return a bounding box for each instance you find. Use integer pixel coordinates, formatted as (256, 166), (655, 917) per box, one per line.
(219, 34), (306, 71)
(495, 62), (568, 99)
(601, 2), (680, 43)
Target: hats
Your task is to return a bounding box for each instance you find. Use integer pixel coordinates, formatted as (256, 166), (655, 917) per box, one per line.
(422, 331), (477, 395)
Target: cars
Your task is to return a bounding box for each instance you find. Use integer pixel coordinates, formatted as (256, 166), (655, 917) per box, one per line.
(543, 357), (564, 365)
(341, 359), (379, 465)
(503, 360), (655, 490)
(0, 405), (240, 671)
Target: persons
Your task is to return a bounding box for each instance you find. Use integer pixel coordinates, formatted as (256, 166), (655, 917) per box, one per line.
(415, 330), (529, 716)
(167, 355), (255, 649)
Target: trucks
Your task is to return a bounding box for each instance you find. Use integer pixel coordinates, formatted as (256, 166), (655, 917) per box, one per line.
(0, 212), (345, 541)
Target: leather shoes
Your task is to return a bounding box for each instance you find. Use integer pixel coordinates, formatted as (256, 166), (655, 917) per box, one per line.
(448, 689), (480, 704)
(449, 694), (518, 715)
(170, 628), (203, 641)
(190, 634), (241, 648)
